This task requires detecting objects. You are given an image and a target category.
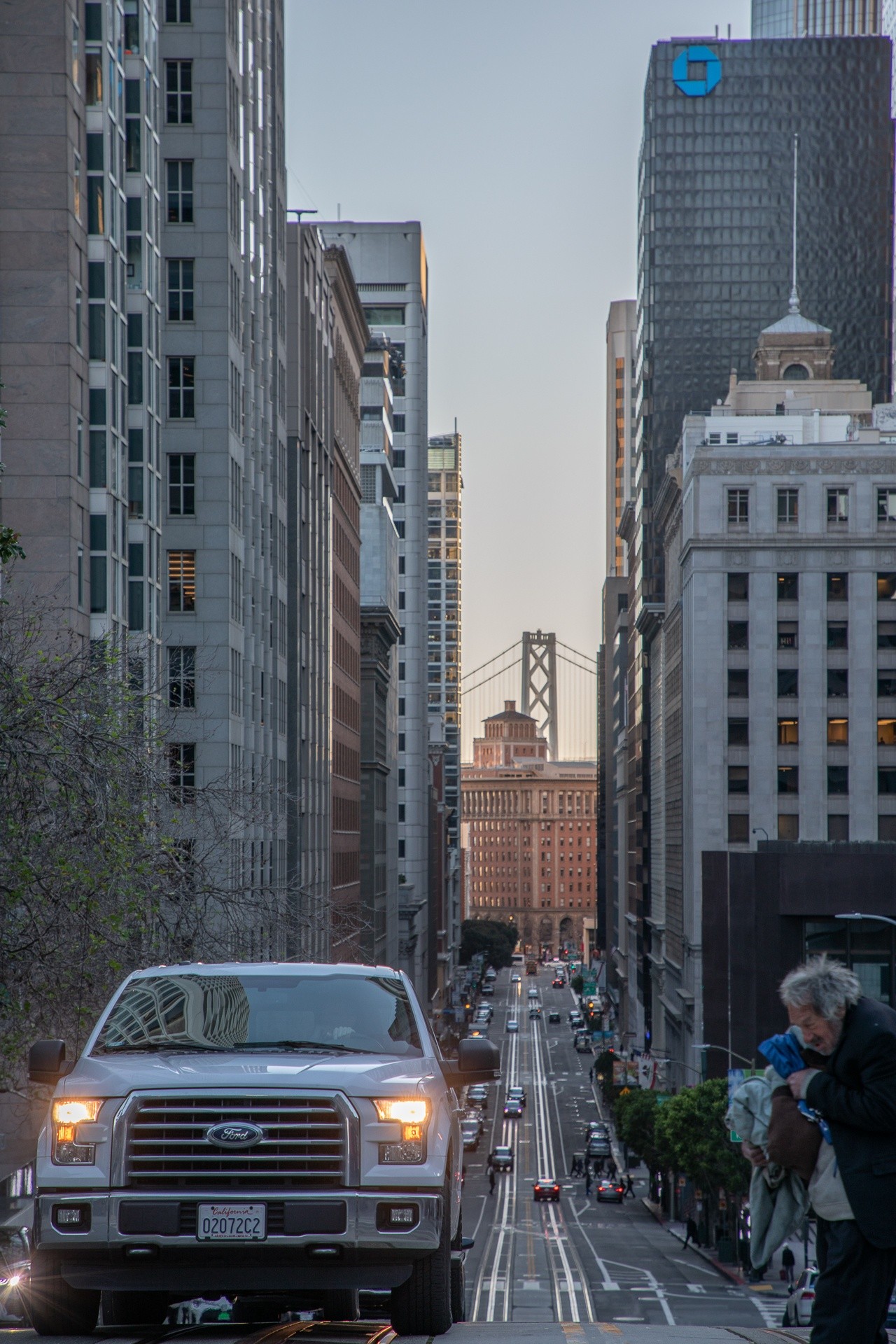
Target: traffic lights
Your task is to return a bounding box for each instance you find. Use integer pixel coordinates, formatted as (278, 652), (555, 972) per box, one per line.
(564, 949), (568, 954)
(570, 963), (575, 969)
(571, 969), (575, 974)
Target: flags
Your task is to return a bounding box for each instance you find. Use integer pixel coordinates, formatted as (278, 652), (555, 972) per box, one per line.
(635, 1050), (657, 1090)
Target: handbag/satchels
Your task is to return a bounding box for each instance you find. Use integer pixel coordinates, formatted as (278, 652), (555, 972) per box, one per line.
(780, 1266), (787, 1281)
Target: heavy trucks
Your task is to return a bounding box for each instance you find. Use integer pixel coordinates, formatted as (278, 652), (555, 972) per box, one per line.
(525, 959), (537, 976)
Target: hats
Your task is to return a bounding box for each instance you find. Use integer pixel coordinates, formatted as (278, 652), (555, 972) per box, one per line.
(782, 1242), (788, 1247)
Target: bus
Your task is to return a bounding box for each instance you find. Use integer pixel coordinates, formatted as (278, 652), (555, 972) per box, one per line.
(511, 955), (524, 967)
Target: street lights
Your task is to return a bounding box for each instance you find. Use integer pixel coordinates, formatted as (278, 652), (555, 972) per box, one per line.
(443, 1028), (480, 1060)
(690, 1042), (757, 1082)
(752, 825), (769, 852)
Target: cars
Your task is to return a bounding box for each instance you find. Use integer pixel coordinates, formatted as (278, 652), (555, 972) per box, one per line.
(511, 974), (520, 982)
(596, 1180), (624, 1203)
(459, 1130), (478, 1150)
(543, 960), (566, 968)
(1, 1224), (33, 1328)
(502, 1100), (523, 1119)
(531, 1178), (561, 1202)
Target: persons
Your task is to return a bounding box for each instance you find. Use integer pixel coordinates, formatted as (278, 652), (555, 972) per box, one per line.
(570, 1153), (637, 1198)
(589, 1067), (594, 1084)
(679, 1213), (702, 1250)
(739, 950), (896, 1344)
(314, 991), (385, 1049)
(620, 1042), (624, 1056)
(486, 1154), (495, 1175)
(489, 1170), (496, 1195)
(781, 1241), (795, 1283)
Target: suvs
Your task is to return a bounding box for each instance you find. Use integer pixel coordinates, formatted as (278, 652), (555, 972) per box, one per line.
(505, 1086), (528, 1107)
(490, 1145), (515, 1170)
(548, 1012), (561, 1023)
(460, 1111), (484, 1133)
(780, 1266), (821, 1327)
(527, 988), (539, 998)
(569, 1011), (581, 1019)
(554, 966), (563, 972)
(571, 1018), (583, 1028)
(467, 1029), (489, 1041)
(23, 957), (501, 1338)
(467, 1082), (485, 1092)
(482, 984), (493, 995)
(552, 978), (564, 988)
(587, 1128), (609, 1141)
(506, 1019), (519, 1032)
(529, 1008), (541, 1019)
(586, 1138), (611, 1157)
(463, 1088), (490, 1108)
(586, 1120), (606, 1132)
(557, 975), (567, 983)
(575, 1035), (593, 1053)
(478, 1004), (493, 1015)
(485, 972), (496, 981)
(476, 1011), (489, 1020)
(473, 1018), (488, 1024)
(556, 971), (565, 976)
